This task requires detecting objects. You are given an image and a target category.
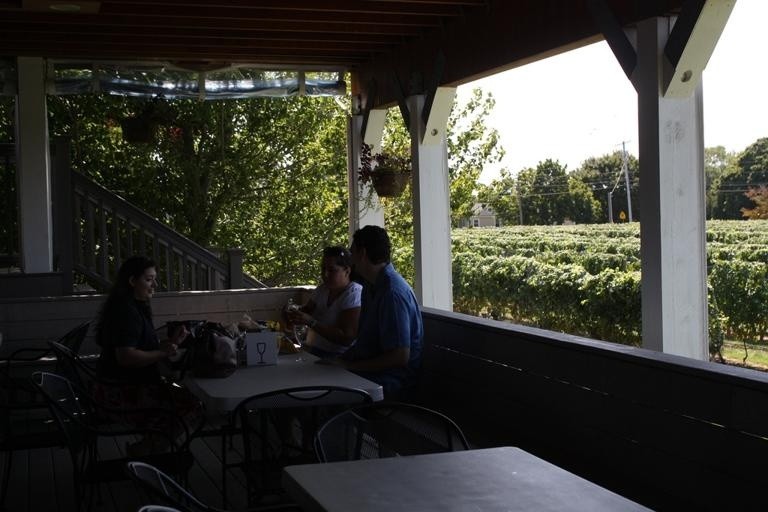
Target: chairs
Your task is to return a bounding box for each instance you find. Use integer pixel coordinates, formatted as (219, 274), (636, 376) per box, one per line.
(30, 371), (194, 511)
(124, 461), (217, 511)
(1, 319), (92, 393)
(234, 386), (374, 509)
(44, 342), (193, 510)
(311, 401), (473, 464)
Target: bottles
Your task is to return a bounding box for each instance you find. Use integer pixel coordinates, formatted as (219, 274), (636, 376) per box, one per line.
(285, 299), (299, 333)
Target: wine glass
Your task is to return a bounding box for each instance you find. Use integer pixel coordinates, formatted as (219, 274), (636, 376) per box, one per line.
(294, 325), (310, 362)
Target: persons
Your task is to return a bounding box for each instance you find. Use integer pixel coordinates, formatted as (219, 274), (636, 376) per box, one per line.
(306, 226), (424, 401)
(285, 243), (362, 363)
(90, 255), (187, 384)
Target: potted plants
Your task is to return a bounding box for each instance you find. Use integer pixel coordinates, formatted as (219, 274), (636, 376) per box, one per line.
(356, 132), (412, 198)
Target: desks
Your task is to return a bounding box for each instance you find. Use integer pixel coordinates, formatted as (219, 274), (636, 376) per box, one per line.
(279, 445), (657, 512)
(176, 340), (385, 509)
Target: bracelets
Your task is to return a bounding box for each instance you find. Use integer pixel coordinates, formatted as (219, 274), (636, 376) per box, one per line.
(308, 318), (318, 328)
